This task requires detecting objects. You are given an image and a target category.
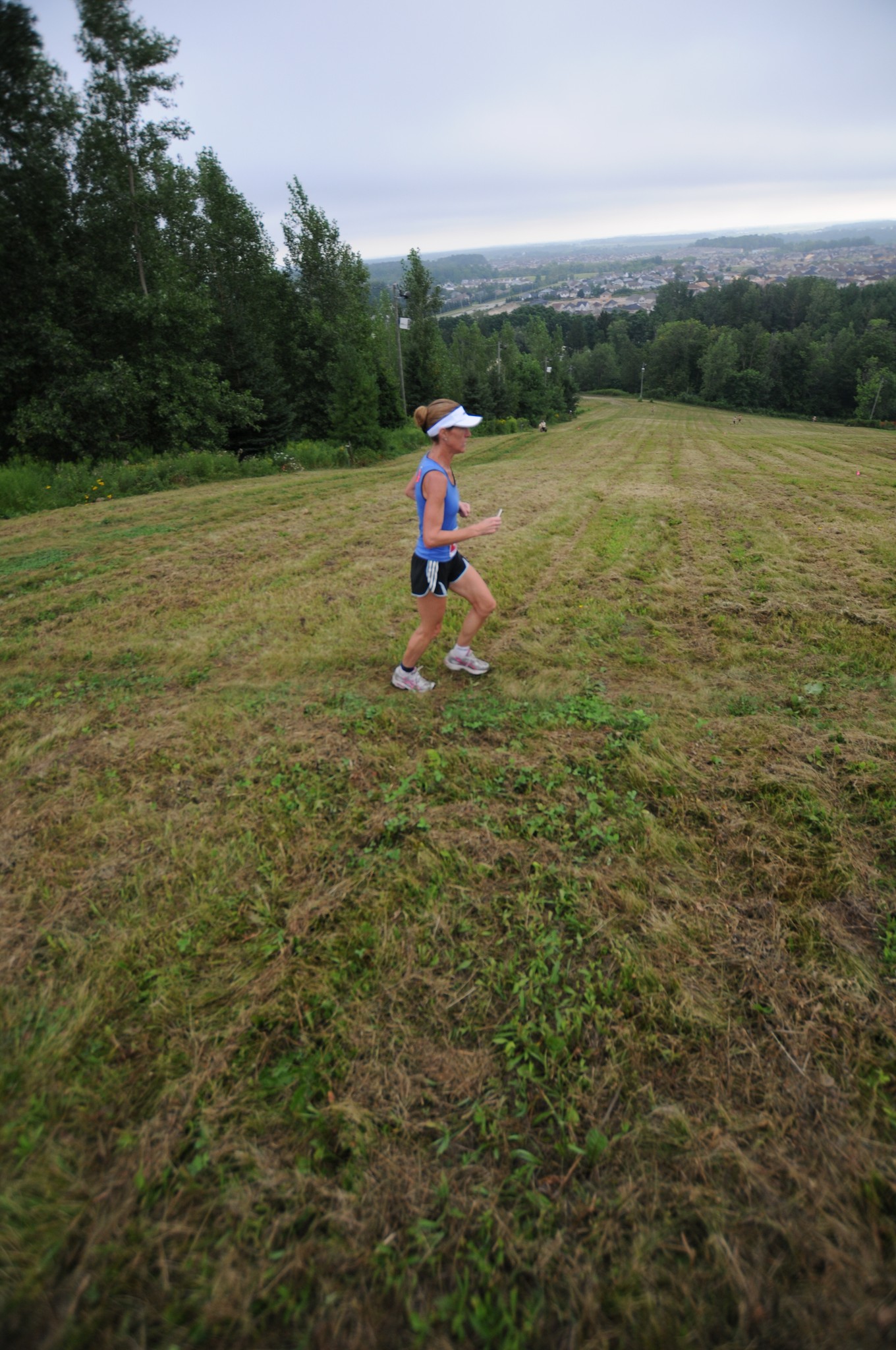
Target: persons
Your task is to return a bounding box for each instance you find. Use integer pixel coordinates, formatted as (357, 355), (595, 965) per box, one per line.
(729, 415), (743, 425)
(391, 398), (503, 694)
(651, 406), (655, 413)
(538, 419), (547, 432)
(812, 416), (817, 423)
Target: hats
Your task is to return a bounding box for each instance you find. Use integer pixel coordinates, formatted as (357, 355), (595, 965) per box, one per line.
(427, 405), (483, 437)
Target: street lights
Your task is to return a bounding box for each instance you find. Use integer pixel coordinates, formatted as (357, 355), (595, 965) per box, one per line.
(639, 362), (647, 398)
(392, 283), (411, 415)
(498, 341), (508, 382)
(545, 356), (553, 387)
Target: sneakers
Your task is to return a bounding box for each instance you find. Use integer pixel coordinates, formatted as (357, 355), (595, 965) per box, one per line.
(444, 647), (489, 675)
(391, 664), (436, 692)
